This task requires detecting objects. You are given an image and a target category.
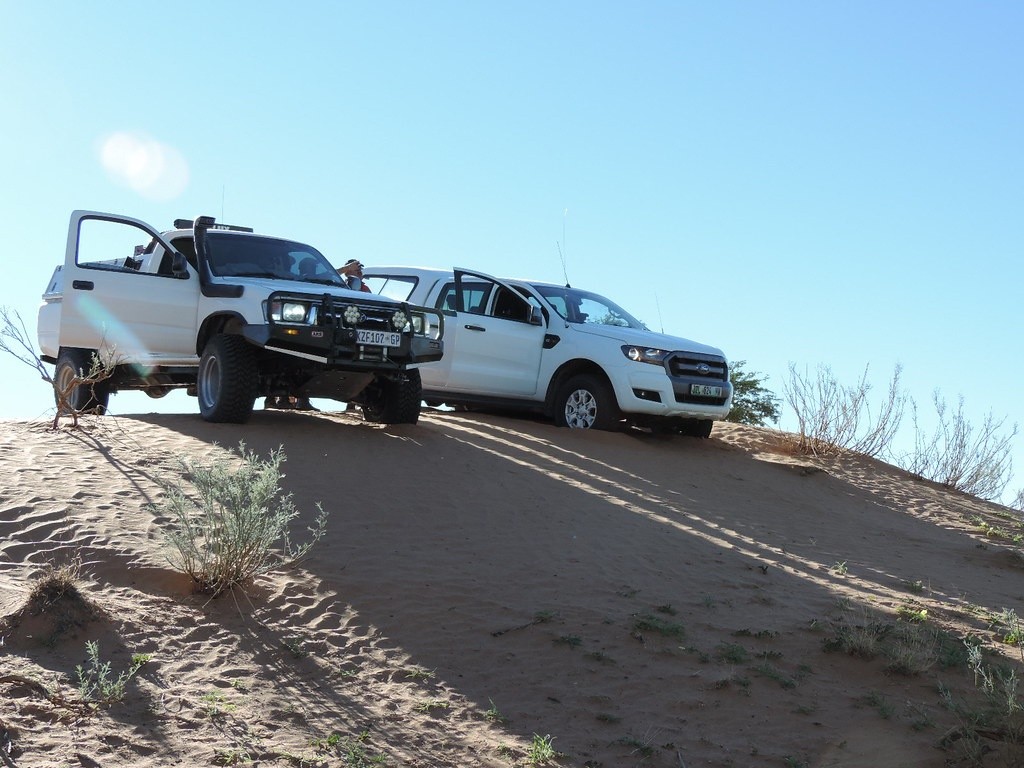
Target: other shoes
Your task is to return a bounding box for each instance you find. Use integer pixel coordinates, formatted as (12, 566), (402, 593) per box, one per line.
(263, 396), (355, 411)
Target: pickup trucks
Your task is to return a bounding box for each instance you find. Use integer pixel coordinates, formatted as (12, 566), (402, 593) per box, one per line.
(37, 208), (446, 423)
(357, 268), (734, 436)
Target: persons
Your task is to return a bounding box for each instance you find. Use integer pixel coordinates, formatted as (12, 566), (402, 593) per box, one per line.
(294, 256), (359, 410)
(344, 259), (375, 414)
(263, 253), (299, 408)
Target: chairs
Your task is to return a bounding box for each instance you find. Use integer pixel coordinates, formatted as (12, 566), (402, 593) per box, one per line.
(527, 305), (542, 325)
